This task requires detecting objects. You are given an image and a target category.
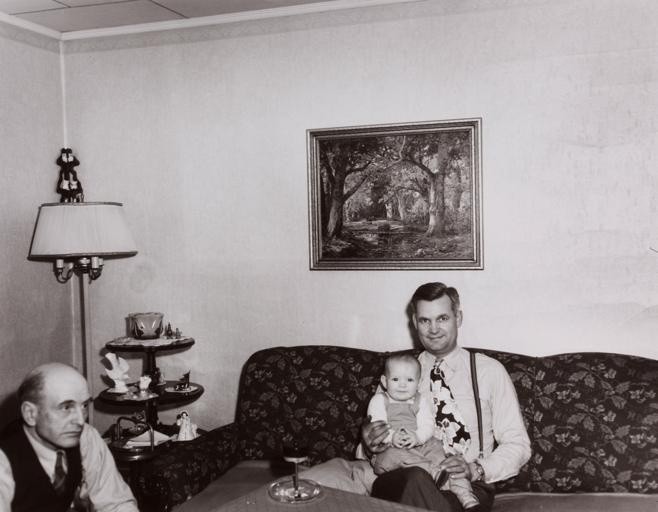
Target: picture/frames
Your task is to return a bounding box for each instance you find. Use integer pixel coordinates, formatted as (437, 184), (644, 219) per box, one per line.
(303, 116), (486, 275)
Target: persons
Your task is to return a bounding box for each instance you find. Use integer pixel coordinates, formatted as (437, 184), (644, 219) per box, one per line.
(0, 361), (140, 512)
(355, 282), (533, 512)
(366, 354), (481, 510)
(174, 411), (199, 443)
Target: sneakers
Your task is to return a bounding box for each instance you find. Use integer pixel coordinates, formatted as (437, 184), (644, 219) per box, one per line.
(458, 492), (479, 509)
(429, 465), (443, 481)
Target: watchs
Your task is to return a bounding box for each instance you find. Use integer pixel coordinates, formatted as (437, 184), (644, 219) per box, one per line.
(474, 461), (485, 481)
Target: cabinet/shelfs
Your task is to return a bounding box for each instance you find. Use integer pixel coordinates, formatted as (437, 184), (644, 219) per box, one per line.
(88, 331), (211, 474)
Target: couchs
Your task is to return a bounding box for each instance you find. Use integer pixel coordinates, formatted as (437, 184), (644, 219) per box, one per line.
(127, 336), (655, 512)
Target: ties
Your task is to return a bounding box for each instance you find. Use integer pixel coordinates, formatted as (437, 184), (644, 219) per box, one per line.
(52, 452), (66, 494)
(430, 358), (471, 455)
